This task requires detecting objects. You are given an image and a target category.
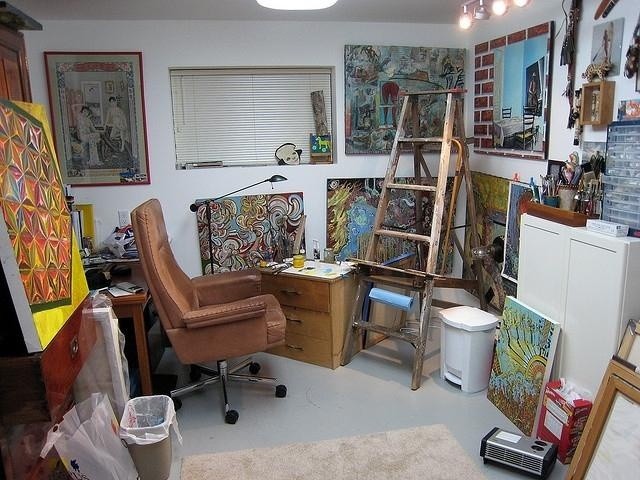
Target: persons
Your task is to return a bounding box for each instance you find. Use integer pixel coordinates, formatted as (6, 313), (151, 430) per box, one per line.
(528, 72), (539, 113)
(105, 96), (128, 153)
(601, 30), (611, 58)
(70, 102), (119, 162)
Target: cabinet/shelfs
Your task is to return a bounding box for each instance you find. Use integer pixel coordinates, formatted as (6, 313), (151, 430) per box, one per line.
(516, 212), (640, 405)
(259, 257), (361, 372)
(1, 0), (98, 479)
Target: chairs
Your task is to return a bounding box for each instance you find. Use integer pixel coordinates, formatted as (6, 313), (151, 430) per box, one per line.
(129, 198), (287, 425)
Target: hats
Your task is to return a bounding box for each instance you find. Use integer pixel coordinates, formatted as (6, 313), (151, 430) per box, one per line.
(532, 72), (536, 77)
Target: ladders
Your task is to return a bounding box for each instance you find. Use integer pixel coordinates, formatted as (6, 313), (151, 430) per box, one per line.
(340, 89), (489, 389)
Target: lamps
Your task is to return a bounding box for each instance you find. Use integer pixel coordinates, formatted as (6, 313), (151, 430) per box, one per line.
(458, 0), (532, 31)
(190, 174), (287, 274)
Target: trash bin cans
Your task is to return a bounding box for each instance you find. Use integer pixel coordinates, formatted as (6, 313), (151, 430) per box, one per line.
(119, 395), (172, 480)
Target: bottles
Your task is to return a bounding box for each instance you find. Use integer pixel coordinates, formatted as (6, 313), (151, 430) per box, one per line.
(293, 255), (304, 268)
(323, 248), (334, 264)
(312, 239), (321, 261)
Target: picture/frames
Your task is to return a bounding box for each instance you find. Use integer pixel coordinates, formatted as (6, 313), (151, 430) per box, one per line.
(44, 50), (151, 187)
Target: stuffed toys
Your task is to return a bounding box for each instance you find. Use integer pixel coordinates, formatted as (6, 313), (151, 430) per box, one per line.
(581, 58), (612, 83)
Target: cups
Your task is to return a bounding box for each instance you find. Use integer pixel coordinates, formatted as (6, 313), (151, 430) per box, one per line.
(543, 195), (559, 208)
(558, 184), (579, 211)
(260, 260), (266, 268)
